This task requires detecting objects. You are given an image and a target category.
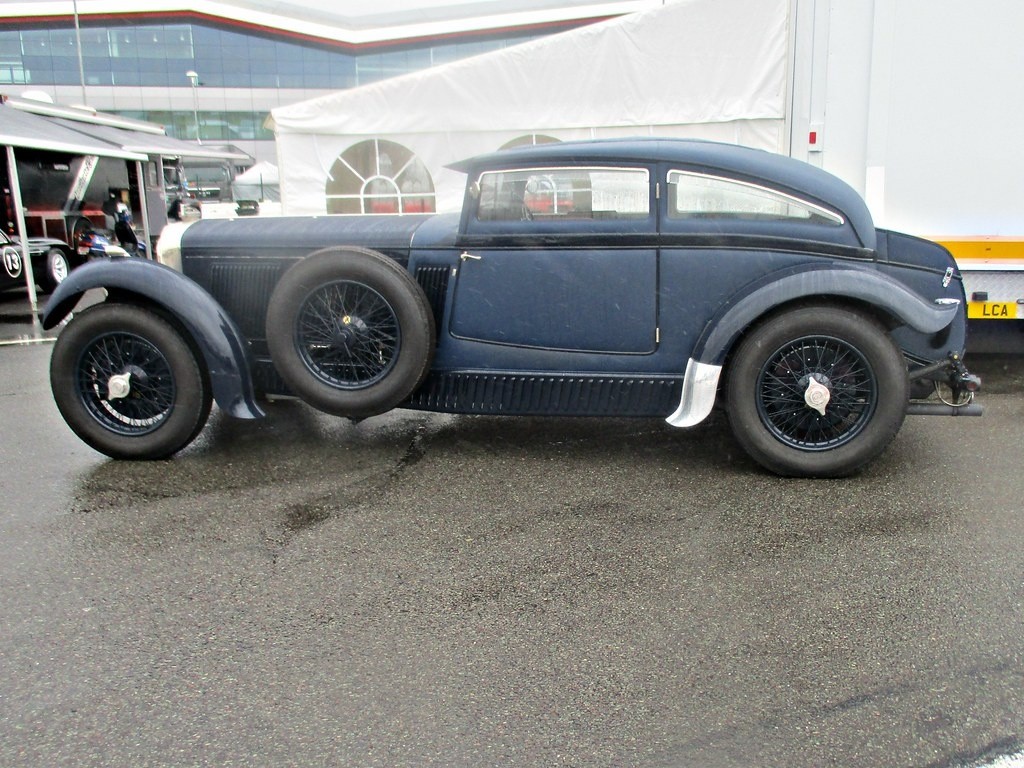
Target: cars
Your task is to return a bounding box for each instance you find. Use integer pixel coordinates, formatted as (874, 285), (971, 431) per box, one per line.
(35, 137), (984, 479)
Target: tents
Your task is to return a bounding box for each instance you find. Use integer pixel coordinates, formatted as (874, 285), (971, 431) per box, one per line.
(232, 159), (280, 202)
